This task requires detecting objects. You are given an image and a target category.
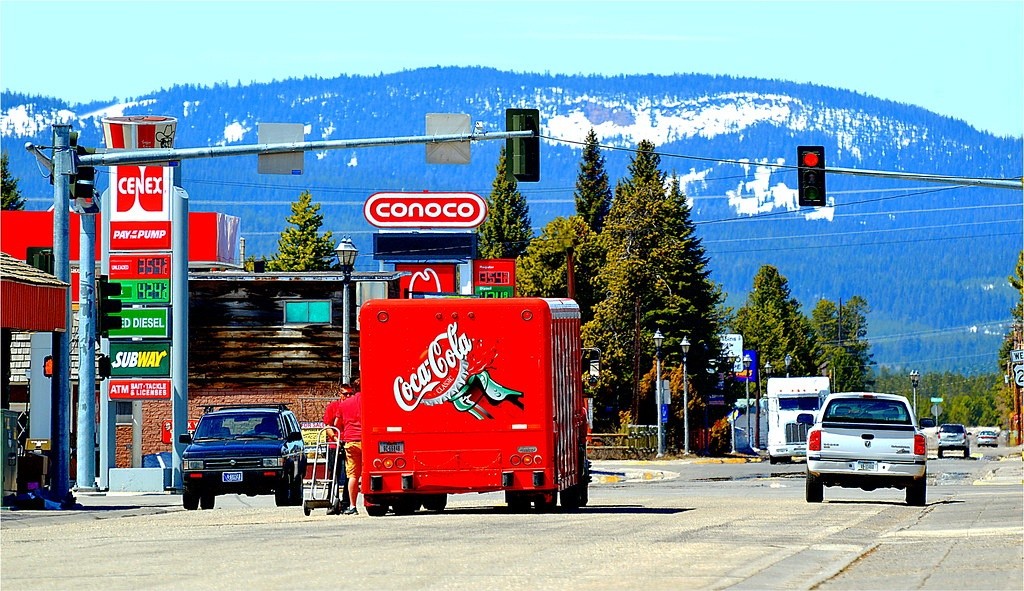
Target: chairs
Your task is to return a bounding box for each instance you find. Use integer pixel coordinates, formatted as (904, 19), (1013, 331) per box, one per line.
(254, 423), (279, 436)
(882, 404), (899, 421)
(832, 405), (853, 418)
(220, 427), (231, 435)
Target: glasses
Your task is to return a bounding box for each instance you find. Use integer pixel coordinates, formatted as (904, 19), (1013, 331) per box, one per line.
(341, 392), (353, 396)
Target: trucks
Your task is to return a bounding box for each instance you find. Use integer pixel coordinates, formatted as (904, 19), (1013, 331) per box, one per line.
(767, 376), (830, 465)
(359, 295), (592, 518)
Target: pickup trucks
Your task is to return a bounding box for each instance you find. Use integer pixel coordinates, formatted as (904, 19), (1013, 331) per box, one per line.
(796, 391), (936, 506)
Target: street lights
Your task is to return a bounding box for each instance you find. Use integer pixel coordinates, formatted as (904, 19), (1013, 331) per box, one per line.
(333, 234), (359, 386)
(743, 355), (752, 448)
(764, 354), (791, 449)
(680, 336), (691, 456)
(652, 328), (666, 459)
(910, 369), (920, 417)
(727, 352), (738, 454)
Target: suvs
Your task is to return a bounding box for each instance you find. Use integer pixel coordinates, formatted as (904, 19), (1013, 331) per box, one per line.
(178, 402), (308, 512)
(935, 423), (972, 457)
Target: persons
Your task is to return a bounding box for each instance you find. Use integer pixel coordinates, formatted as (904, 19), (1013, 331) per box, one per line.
(259, 416), (283, 440)
(324, 378), (362, 516)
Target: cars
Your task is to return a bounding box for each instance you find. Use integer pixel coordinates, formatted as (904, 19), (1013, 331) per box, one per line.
(976, 430), (999, 448)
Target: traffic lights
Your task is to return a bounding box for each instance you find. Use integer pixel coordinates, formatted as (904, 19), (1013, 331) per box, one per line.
(797, 145), (826, 207)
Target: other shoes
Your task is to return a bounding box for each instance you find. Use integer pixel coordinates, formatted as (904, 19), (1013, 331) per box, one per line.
(344, 506), (357, 514)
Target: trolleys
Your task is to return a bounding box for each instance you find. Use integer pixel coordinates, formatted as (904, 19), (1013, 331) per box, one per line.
(303, 425), (346, 516)
